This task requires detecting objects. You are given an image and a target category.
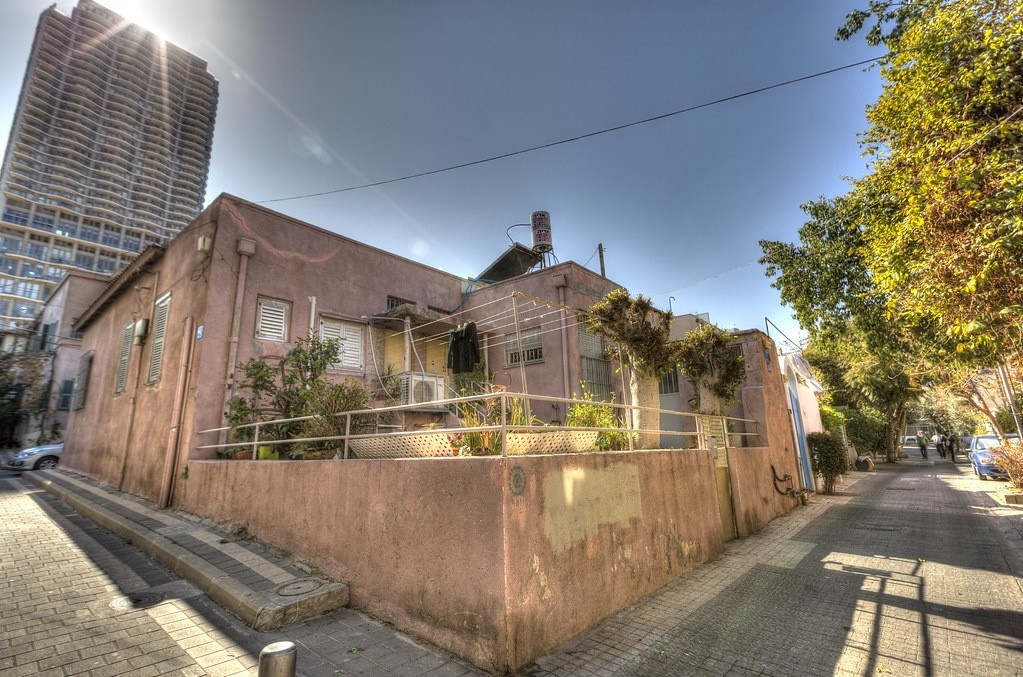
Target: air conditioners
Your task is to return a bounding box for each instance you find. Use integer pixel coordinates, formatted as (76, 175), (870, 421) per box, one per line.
(401, 376), (444, 408)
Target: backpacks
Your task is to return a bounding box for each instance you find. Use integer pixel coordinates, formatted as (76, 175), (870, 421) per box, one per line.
(944, 435), (950, 446)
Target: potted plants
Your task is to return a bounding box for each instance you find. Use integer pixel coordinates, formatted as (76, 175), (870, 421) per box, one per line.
(236, 442), (254, 460)
(257, 435), (279, 460)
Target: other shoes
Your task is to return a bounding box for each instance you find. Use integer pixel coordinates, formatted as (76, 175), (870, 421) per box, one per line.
(923, 457), (928, 459)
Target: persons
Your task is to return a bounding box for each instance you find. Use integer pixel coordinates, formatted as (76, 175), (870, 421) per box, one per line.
(941, 430), (955, 463)
(917, 431), (928, 459)
(931, 432), (946, 459)
(962, 431), (972, 446)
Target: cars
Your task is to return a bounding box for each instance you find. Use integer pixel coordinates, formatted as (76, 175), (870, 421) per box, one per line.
(965, 434), (1022, 480)
(5, 440), (66, 472)
